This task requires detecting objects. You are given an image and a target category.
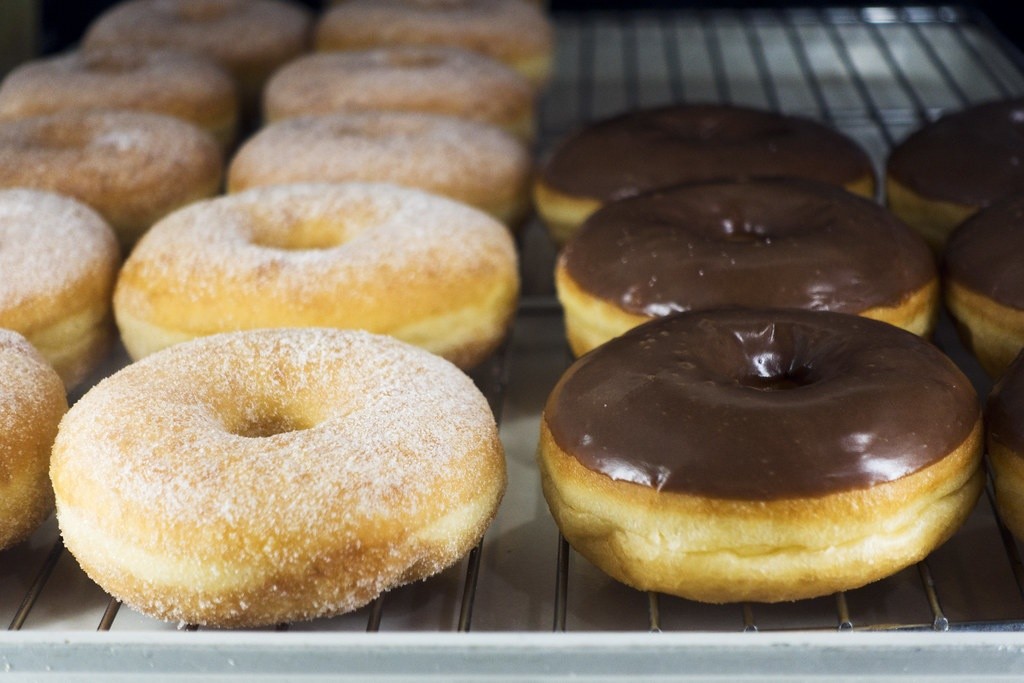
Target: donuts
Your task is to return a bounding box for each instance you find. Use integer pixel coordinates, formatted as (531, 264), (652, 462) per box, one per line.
(0, 0), (561, 628)
(532, 97), (1024, 604)
